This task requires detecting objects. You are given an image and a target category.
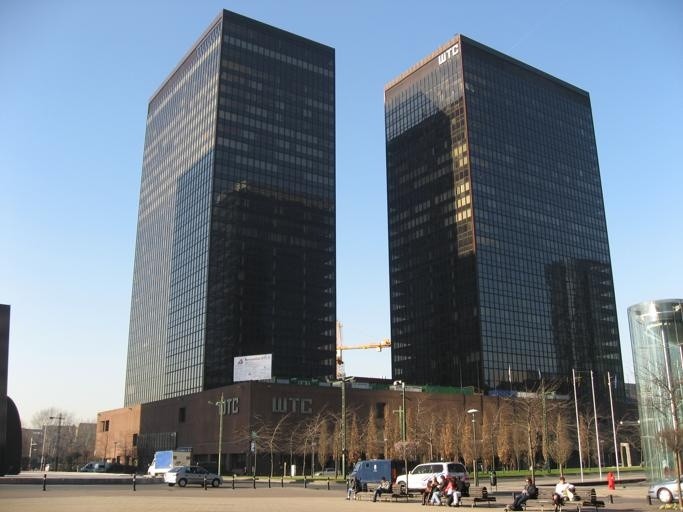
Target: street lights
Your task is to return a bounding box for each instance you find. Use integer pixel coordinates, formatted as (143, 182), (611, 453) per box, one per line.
(207, 392), (226, 476)
(50, 412), (63, 471)
(467, 407), (480, 485)
(325, 375), (357, 480)
(393, 380), (407, 441)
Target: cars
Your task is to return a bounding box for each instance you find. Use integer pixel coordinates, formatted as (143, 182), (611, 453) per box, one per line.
(315, 468), (341, 477)
(80, 463), (107, 472)
(164, 466), (223, 486)
(649, 474), (683, 504)
(396, 462), (467, 492)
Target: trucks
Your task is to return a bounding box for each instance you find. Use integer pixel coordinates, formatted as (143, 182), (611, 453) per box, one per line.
(148, 449), (191, 476)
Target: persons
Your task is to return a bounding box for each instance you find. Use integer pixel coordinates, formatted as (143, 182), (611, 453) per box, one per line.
(373, 477), (390, 502)
(346, 479), (359, 500)
(553, 477), (574, 512)
(508, 478), (537, 510)
(422, 475), (469, 506)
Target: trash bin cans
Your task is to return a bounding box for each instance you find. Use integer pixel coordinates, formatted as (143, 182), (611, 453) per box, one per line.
(360, 480), (368, 493)
(291, 465), (297, 476)
(46, 464), (52, 471)
(490, 470), (497, 492)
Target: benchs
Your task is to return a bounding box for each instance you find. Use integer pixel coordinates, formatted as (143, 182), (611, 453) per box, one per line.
(357, 483), (413, 503)
(512, 485), (606, 512)
(429, 486), (496, 509)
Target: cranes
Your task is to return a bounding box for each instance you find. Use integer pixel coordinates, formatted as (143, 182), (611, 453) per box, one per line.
(336, 321), (391, 377)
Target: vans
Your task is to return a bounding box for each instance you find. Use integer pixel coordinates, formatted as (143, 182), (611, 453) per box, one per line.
(349, 459), (405, 488)
